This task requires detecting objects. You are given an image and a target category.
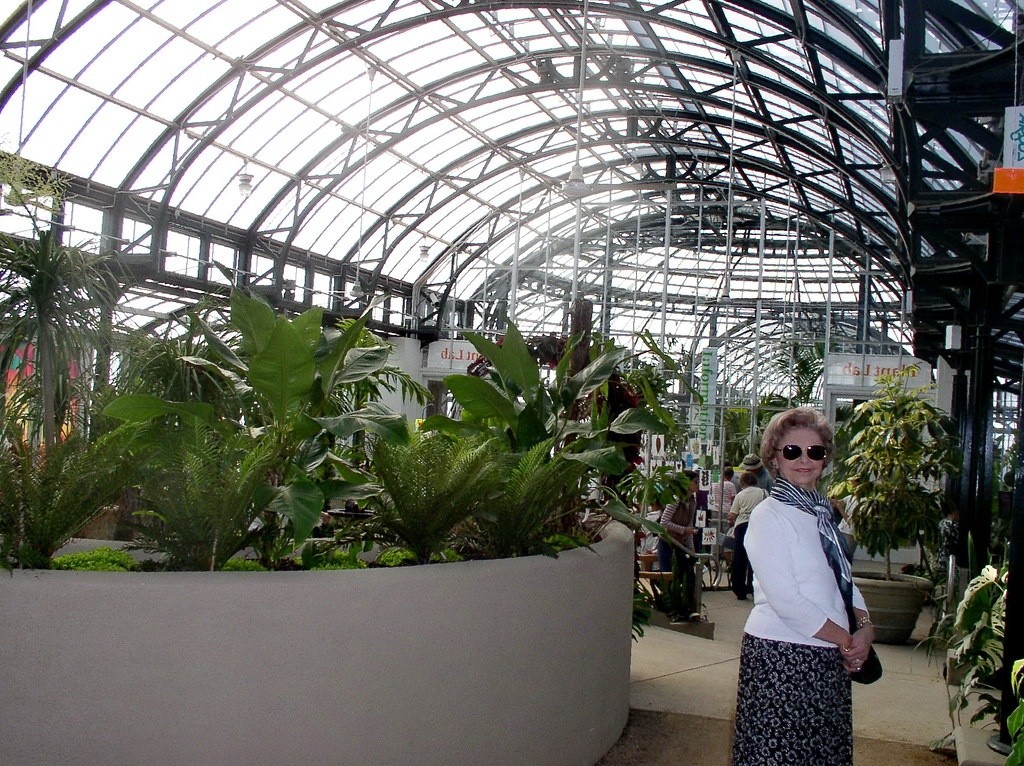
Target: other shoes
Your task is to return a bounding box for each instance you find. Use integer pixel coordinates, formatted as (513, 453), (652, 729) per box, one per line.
(737, 594), (747, 600)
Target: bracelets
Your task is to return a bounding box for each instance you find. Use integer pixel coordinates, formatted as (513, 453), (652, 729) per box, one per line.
(857, 616), (874, 631)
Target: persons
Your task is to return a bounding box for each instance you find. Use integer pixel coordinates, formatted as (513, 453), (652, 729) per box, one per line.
(711, 466), (737, 571)
(739, 453), (772, 493)
(657, 470), (700, 620)
(728, 473), (769, 601)
(730, 407), (875, 765)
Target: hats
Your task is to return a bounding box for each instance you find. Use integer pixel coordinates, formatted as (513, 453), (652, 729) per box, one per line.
(739, 453), (762, 469)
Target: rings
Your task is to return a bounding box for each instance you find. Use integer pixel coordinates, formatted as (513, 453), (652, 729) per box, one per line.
(851, 657), (862, 672)
(842, 644), (851, 651)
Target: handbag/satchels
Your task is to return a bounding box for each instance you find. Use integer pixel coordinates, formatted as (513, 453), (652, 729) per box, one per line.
(850, 629), (921, 726)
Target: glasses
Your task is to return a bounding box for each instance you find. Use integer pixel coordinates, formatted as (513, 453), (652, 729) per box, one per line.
(695, 480), (700, 485)
(773, 445), (828, 461)
